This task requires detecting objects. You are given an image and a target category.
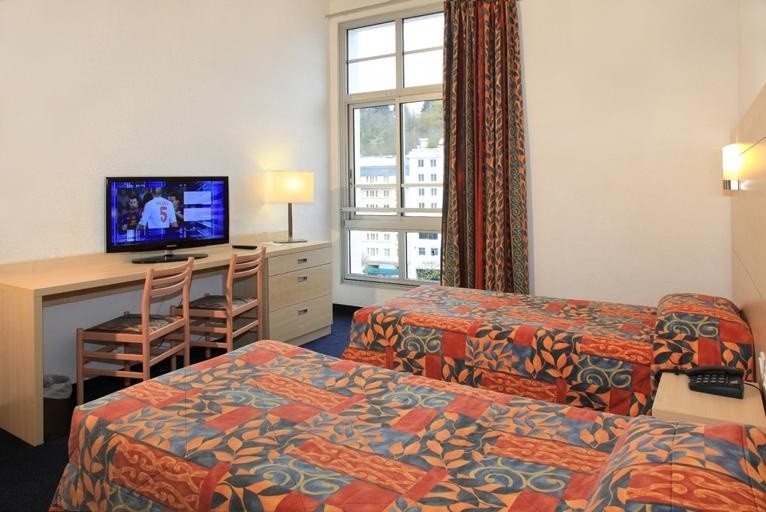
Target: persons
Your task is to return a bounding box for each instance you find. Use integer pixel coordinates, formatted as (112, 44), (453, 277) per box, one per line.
(116, 187), (184, 231)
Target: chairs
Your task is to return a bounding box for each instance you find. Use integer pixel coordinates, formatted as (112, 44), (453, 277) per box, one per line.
(75, 256), (196, 399)
(171, 245), (267, 364)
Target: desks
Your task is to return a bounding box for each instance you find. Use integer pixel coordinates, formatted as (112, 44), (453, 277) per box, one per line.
(1, 233), (332, 447)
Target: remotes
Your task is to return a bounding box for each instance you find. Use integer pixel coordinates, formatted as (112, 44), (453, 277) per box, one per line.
(232, 245), (257, 249)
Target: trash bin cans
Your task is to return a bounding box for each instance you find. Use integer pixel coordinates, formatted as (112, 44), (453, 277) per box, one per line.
(44, 375), (72, 440)
(686, 366), (744, 398)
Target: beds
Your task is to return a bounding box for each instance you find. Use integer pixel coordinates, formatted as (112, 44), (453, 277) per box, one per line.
(342, 285), (766, 416)
(42, 341), (766, 512)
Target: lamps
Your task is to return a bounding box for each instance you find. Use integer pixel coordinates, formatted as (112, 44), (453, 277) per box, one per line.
(266, 170), (313, 243)
(722, 143), (740, 192)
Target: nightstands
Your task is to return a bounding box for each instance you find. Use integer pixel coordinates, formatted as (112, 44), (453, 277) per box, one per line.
(650, 365), (766, 430)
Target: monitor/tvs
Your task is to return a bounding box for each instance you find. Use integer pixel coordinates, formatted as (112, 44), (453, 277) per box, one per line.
(105, 176), (230, 264)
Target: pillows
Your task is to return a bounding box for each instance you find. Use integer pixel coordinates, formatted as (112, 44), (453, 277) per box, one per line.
(646, 291), (758, 375)
(589, 415), (766, 512)
